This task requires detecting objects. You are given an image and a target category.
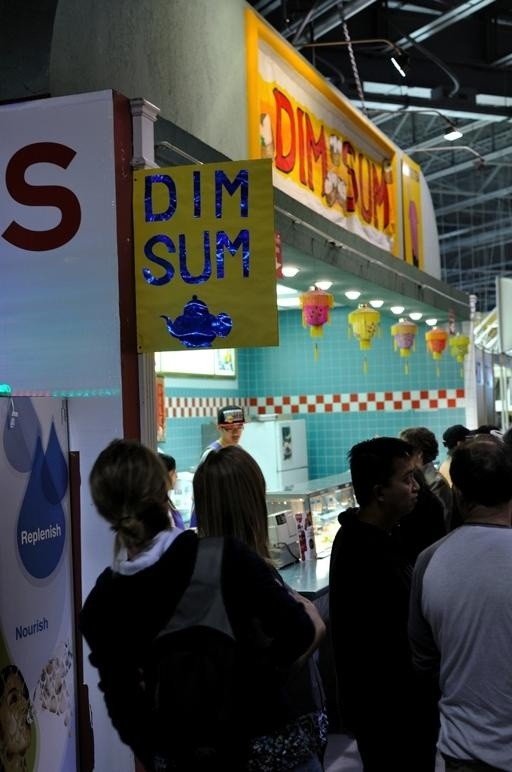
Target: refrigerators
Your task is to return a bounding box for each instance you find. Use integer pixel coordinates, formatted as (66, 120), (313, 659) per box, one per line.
(214, 418), (308, 492)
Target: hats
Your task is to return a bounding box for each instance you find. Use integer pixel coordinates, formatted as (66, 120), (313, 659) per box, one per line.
(218, 405), (245, 425)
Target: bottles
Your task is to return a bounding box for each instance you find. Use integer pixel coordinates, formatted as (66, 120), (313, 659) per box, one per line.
(305, 528), (316, 561)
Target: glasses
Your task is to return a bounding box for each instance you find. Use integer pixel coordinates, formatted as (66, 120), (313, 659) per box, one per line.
(222, 426), (245, 432)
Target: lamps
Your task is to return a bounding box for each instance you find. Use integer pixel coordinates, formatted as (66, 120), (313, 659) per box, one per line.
(402, 145), (486, 170)
(293, 38), (414, 78)
(364, 108), (464, 142)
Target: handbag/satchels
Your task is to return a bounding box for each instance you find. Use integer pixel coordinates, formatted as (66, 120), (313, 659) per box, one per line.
(141, 536), (252, 753)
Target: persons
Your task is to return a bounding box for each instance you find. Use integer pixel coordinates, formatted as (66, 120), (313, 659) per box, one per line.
(189, 405), (245, 528)
(0, 661), (36, 772)
(157, 452), (186, 532)
(78, 437), (327, 772)
(401, 424), (511, 568)
(191, 445), (326, 772)
(328, 438), (442, 772)
(406, 434), (510, 772)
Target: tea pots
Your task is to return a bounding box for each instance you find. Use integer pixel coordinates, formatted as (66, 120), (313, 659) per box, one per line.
(159, 295), (232, 349)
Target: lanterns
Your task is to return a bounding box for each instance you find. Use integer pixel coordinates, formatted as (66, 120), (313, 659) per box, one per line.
(347, 302), (385, 374)
(449, 334), (470, 377)
(424, 326), (449, 378)
(390, 318), (419, 376)
(299, 286), (334, 363)
